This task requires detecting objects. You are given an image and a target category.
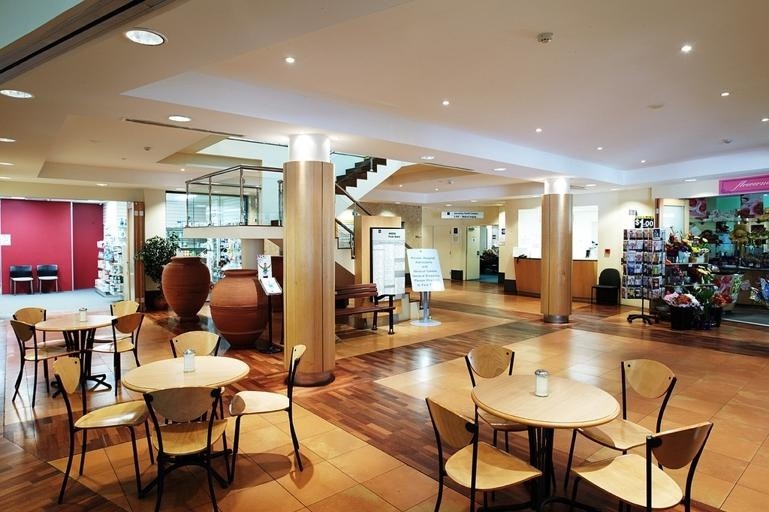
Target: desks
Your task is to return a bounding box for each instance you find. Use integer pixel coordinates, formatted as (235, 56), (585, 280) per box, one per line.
(122, 356), (251, 496)
(471, 375), (620, 512)
(35, 314), (119, 398)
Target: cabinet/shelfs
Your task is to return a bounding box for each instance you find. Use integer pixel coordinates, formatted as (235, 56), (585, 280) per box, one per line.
(664, 243), (715, 330)
(94, 239), (124, 297)
(622, 227), (666, 325)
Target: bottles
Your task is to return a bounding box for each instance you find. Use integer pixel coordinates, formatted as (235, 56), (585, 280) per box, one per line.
(182, 347), (196, 373)
(78, 307), (88, 321)
(534, 369), (551, 397)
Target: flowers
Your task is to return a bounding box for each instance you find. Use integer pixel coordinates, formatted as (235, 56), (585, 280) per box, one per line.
(661, 226), (744, 320)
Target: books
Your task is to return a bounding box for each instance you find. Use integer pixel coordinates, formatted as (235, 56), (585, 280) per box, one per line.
(260, 277), (282, 296)
(257, 254), (272, 277)
(621, 229), (667, 298)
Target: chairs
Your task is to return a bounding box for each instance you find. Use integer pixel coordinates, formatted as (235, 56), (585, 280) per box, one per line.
(564, 358), (677, 488)
(37, 264), (59, 294)
(571, 420), (714, 512)
(52, 357), (154, 504)
(170, 331), (225, 418)
(425, 396), (542, 512)
(591, 268), (621, 307)
(229, 344), (307, 481)
(9, 265), (34, 296)
(464, 344), (531, 453)
(86, 312), (141, 396)
(94, 301), (140, 368)
(13, 306), (66, 378)
(143, 386), (228, 512)
(10, 320), (80, 408)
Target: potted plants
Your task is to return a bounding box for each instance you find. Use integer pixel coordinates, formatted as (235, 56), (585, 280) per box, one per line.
(133, 232), (180, 309)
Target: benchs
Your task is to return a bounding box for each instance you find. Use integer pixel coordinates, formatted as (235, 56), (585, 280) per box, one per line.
(335, 283), (396, 335)
